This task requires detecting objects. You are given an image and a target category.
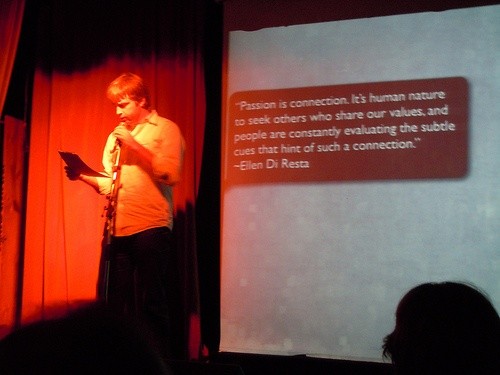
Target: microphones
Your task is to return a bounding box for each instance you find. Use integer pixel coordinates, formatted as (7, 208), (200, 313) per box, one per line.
(109, 122), (127, 153)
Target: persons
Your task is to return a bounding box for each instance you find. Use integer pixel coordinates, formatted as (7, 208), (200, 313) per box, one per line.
(65, 73), (185, 339)
(382, 281), (500, 375)
(1, 298), (190, 375)
